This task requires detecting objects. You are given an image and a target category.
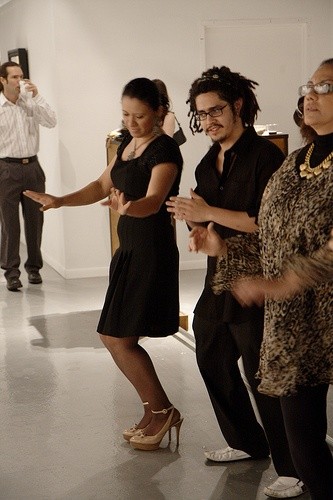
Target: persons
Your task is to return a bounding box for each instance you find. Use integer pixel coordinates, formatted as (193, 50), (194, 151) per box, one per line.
(23, 57), (333, 500)
(0, 62), (57, 290)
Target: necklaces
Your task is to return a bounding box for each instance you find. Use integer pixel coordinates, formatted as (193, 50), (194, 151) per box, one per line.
(300, 144), (333, 178)
(128, 129), (162, 161)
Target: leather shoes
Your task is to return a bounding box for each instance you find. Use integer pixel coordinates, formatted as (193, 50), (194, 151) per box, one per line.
(28, 271), (41, 283)
(7, 277), (23, 289)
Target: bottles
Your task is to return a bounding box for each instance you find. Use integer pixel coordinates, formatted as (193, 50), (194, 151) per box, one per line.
(19, 78), (35, 109)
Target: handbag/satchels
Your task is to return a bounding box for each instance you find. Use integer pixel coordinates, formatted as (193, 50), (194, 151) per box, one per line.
(167, 111), (186, 147)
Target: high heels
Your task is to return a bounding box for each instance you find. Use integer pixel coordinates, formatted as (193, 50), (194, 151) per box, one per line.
(123, 402), (171, 440)
(130, 406), (183, 450)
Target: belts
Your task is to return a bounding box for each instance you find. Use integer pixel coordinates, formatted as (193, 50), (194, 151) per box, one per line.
(0, 156), (37, 164)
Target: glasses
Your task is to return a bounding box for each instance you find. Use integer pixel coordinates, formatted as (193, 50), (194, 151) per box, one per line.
(298, 82), (333, 97)
(195, 97), (238, 121)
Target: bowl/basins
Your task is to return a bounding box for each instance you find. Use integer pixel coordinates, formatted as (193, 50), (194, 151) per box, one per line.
(254, 125), (266, 136)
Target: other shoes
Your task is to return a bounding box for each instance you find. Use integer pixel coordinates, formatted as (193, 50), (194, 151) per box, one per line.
(205, 446), (252, 461)
(263, 476), (304, 498)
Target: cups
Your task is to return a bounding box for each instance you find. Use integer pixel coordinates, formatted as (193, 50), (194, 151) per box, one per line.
(267, 123), (277, 135)
